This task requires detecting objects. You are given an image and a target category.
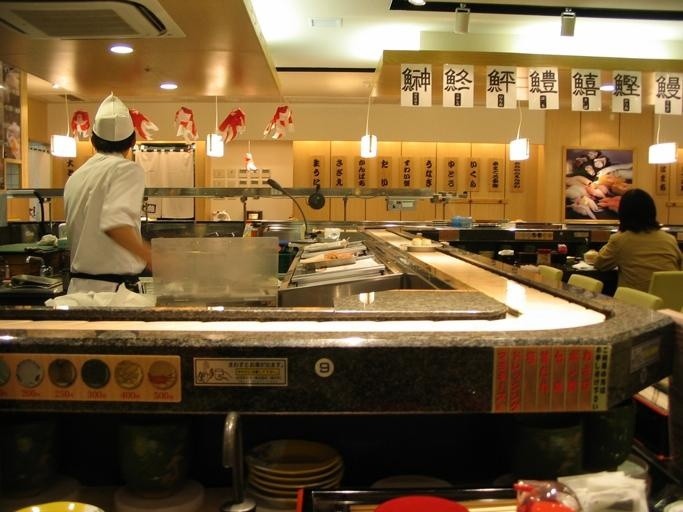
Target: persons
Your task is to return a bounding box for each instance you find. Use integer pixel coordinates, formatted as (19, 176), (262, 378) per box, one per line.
(64, 91), (152, 295)
(592, 189), (683, 294)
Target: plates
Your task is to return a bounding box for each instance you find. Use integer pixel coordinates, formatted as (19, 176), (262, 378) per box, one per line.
(17, 500), (105, 511)
(375, 494), (468, 512)
(245, 439), (343, 505)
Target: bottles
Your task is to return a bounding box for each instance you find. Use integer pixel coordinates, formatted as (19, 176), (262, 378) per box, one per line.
(468, 217), (472, 227)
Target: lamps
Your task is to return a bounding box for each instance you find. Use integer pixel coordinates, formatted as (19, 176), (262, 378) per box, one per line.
(561, 8), (576, 36)
(360, 97), (378, 158)
(509, 100), (530, 161)
(454, 3), (471, 34)
(206, 96), (225, 158)
(648, 115), (677, 164)
(50, 94), (77, 158)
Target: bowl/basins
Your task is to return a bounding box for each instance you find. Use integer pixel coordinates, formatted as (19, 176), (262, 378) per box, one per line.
(460, 222), (470, 228)
(583, 253), (598, 265)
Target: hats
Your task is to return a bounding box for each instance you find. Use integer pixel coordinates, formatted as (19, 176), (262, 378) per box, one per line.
(92, 91), (134, 142)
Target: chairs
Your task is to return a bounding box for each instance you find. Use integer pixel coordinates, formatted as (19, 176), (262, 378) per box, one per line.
(537, 265), (564, 280)
(648, 271), (683, 313)
(613, 286), (664, 311)
(567, 274), (604, 293)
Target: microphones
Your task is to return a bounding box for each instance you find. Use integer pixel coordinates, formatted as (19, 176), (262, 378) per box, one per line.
(264, 178), (309, 239)
